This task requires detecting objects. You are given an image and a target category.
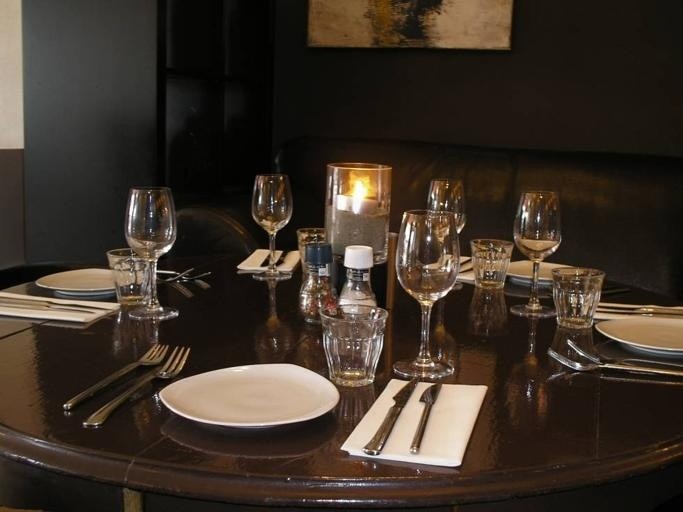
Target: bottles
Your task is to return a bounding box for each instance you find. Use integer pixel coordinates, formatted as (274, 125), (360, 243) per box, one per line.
(300, 244), (336, 323)
(336, 246), (378, 316)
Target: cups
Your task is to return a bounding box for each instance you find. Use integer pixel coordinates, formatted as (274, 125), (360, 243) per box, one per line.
(297, 228), (328, 279)
(320, 304), (386, 387)
(113, 309), (159, 356)
(331, 386), (380, 462)
(426, 179), (466, 242)
(108, 249), (154, 306)
(552, 266), (605, 327)
(469, 288), (508, 342)
(470, 239), (511, 291)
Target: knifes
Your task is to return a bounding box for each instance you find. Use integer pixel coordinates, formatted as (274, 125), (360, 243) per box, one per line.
(260, 249), (289, 268)
(596, 305), (682, 312)
(0, 296), (113, 314)
(364, 375), (419, 457)
(408, 383), (441, 454)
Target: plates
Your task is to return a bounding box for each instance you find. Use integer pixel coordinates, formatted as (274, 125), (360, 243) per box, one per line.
(505, 260), (574, 284)
(159, 415), (336, 460)
(596, 316), (682, 358)
(35, 266), (135, 296)
(162, 360), (344, 431)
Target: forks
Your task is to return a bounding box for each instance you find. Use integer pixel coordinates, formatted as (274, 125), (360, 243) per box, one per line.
(83, 346), (191, 426)
(568, 339), (683, 368)
(547, 347), (683, 374)
(156, 266), (212, 283)
(545, 370), (682, 387)
(61, 342), (170, 411)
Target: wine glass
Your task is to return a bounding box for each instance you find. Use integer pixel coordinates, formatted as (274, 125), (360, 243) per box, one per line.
(249, 174), (292, 282)
(250, 284), (294, 361)
(513, 190), (562, 318)
(496, 315), (553, 436)
(393, 208), (457, 378)
(125, 186), (181, 320)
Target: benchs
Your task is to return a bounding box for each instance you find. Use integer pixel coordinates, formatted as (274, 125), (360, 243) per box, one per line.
(134, 121), (681, 312)
